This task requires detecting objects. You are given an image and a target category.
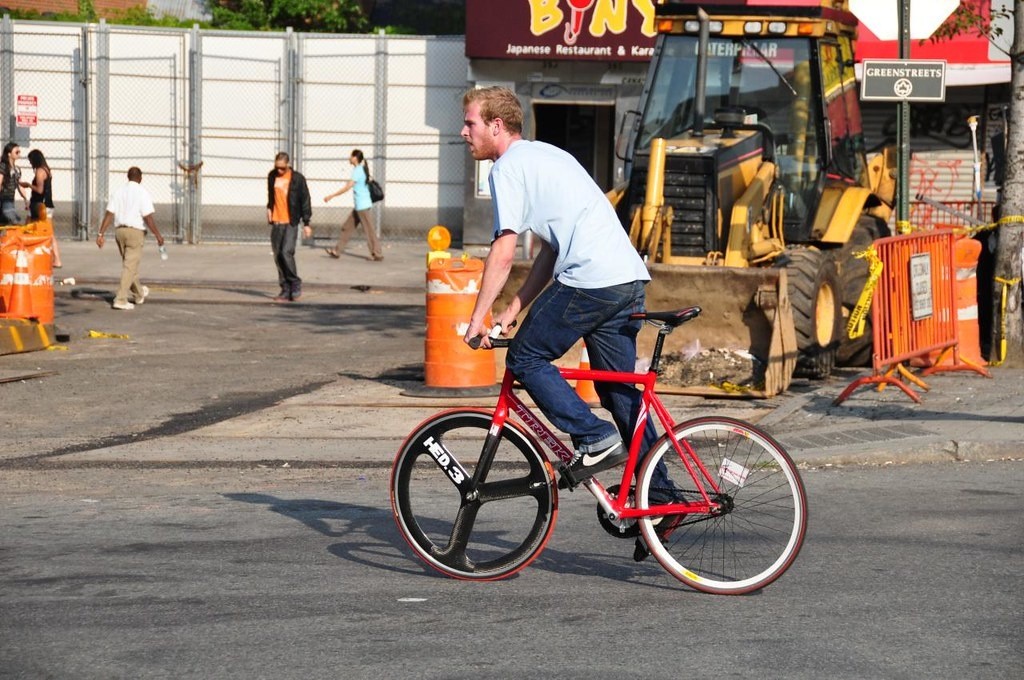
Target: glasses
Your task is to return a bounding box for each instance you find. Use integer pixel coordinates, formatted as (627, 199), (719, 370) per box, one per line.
(10, 150), (21, 155)
(274, 165), (287, 171)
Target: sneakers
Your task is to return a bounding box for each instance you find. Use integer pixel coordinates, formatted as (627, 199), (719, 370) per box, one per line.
(112, 302), (134, 310)
(559, 441), (629, 490)
(633, 497), (689, 561)
(135, 285), (149, 304)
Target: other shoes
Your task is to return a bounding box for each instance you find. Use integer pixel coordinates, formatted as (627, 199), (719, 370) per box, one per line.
(324, 247), (341, 259)
(373, 256), (384, 265)
(292, 285), (302, 302)
(274, 292), (290, 301)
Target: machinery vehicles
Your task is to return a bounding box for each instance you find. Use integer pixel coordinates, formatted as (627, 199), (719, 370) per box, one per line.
(493, 6), (902, 399)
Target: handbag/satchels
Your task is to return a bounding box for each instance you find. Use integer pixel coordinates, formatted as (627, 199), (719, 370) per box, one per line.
(364, 168), (384, 202)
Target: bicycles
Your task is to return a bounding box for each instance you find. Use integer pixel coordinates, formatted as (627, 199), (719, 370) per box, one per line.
(390, 307), (809, 596)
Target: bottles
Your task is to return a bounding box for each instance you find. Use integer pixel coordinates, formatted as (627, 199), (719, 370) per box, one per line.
(159, 245), (168, 260)
(60, 276), (75, 286)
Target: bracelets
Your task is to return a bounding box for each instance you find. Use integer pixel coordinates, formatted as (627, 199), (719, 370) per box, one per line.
(97, 233), (102, 236)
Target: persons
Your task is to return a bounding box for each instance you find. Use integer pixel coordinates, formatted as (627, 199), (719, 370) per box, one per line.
(266, 152), (313, 301)
(324, 150), (385, 262)
(96, 167), (164, 311)
(0, 142), (63, 269)
(460, 85), (688, 562)
